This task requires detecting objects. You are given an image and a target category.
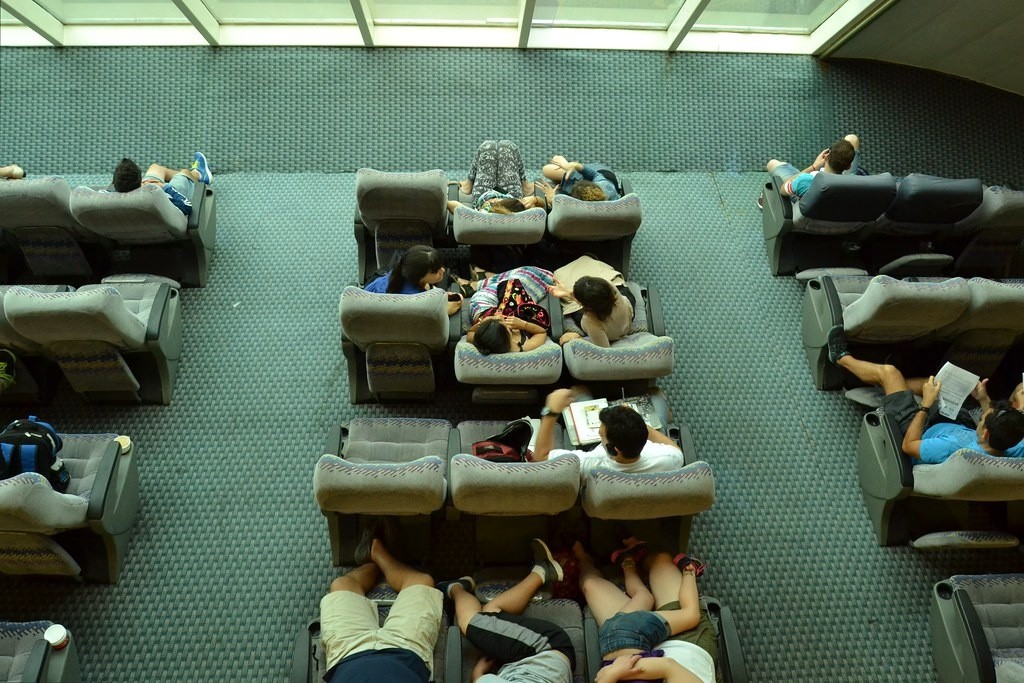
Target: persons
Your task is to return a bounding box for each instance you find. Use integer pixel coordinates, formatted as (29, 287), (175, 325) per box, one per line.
(533, 154), (621, 209)
(533, 387), (684, 487)
(447, 140), (546, 216)
(318, 519), (445, 683)
(756, 134), (861, 206)
(0, 347), (18, 392)
(0, 164), (27, 180)
(570, 533), (720, 683)
(98, 150), (214, 216)
(546, 253), (636, 348)
(449, 263), (554, 357)
(436, 537), (576, 683)
(363, 244), (464, 316)
(827, 324), (1024, 465)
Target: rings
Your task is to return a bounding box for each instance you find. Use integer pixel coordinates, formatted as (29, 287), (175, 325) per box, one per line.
(504, 324), (508, 327)
(543, 185), (546, 188)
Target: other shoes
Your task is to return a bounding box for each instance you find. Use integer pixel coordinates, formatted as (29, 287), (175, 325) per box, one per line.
(758, 198), (762, 208)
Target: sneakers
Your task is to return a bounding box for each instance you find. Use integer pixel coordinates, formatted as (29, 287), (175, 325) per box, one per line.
(884, 352), (904, 376)
(532, 538), (563, 585)
(0, 349), (17, 392)
(827, 324), (850, 366)
(189, 152), (213, 184)
(436, 577), (475, 600)
(673, 553), (704, 577)
(351, 516), (396, 567)
(611, 540), (649, 565)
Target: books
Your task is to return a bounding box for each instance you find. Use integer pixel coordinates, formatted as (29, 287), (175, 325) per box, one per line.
(560, 393), (663, 446)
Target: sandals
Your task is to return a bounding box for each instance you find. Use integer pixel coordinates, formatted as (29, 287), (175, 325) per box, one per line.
(450, 273), (476, 297)
(468, 264), (487, 280)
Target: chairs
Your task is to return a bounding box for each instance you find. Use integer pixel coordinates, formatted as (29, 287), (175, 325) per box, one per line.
(0, 175), (216, 683)
(762, 171), (1024, 683)
(290, 169), (749, 683)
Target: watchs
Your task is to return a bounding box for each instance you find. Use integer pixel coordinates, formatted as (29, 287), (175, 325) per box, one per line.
(540, 407), (562, 417)
(547, 203), (552, 209)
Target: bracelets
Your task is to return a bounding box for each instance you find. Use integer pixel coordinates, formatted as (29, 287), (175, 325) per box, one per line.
(810, 164), (816, 171)
(919, 407), (930, 413)
(534, 195), (538, 205)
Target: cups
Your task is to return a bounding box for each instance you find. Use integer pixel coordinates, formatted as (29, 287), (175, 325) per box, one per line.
(113, 435), (130, 454)
(43, 624), (69, 650)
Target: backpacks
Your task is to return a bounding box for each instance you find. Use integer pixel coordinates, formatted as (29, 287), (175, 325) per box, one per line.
(472, 419), (534, 462)
(0, 415), (70, 493)
